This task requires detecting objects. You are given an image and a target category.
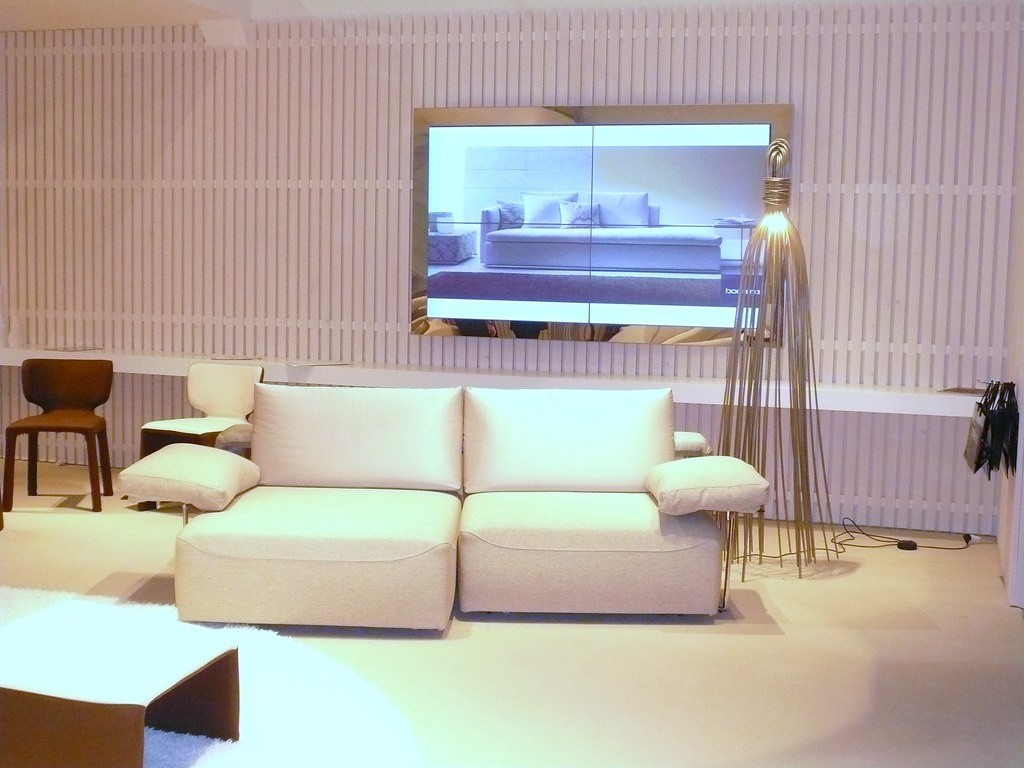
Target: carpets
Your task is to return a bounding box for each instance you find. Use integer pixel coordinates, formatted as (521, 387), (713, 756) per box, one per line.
(0, 588), (421, 768)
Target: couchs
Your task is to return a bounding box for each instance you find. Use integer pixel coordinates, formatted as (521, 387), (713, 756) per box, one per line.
(115, 382), (778, 631)
(479, 191), (721, 272)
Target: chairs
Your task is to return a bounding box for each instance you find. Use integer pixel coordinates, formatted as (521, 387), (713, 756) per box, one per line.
(137, 362), (264, 511)
(2, 359), (115, 513)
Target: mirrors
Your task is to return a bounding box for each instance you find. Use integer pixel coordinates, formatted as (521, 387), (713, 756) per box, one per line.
(715, 221), (761, 268)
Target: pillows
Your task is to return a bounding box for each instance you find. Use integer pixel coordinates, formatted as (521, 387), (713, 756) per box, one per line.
(496, 199), (525, 230)
(521, 193), (579, 229)
(559, 202), (601, 228)
